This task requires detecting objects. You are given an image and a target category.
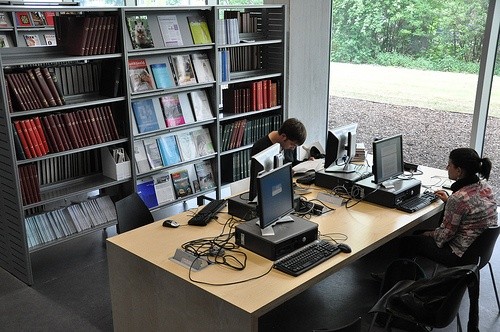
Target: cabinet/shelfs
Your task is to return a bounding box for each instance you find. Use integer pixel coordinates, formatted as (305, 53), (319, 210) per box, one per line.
(0, 4), (288, 286)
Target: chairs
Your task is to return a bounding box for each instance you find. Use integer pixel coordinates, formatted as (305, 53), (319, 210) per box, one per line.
(313, 226), (500, 332)
(115, 192), (154, 233)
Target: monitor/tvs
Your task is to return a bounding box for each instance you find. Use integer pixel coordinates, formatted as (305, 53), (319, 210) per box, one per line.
(324, 123), (358, 174)
(371, 134), (410, 188)
(248, 143), (296, 237)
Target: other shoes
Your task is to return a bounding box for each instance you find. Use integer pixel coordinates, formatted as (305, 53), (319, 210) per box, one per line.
(367, 271), (386, 284)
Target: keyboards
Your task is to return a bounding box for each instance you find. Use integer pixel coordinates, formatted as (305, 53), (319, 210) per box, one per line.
(188, 200), (228, 226)
(396, 191), (438, 213)
(296, 173), (316, 185)
(272, 240), (341, 277)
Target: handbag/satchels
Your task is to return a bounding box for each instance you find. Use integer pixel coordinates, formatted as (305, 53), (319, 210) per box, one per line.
(388, 269), (468, 323)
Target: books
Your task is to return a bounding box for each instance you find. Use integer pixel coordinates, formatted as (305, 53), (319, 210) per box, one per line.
(0, 0), (284, 249)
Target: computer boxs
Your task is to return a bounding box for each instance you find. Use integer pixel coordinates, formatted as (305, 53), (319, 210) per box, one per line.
(227, 191), (318, 261)
(315, 166), (422, 209)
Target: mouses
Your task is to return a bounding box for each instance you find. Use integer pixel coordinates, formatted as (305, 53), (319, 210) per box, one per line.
(162, 220), (179, 228)
(335, 243), (351, 253)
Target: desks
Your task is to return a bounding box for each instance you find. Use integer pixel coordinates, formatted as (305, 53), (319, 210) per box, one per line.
(108, 153), (457, 332)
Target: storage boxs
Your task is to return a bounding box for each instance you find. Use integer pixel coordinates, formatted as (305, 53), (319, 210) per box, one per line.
(101, 147), (132, 181)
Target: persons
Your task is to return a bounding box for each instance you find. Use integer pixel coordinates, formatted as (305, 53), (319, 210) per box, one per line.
(372, 147), (498, 283)
(250, 118), (315, 168)
